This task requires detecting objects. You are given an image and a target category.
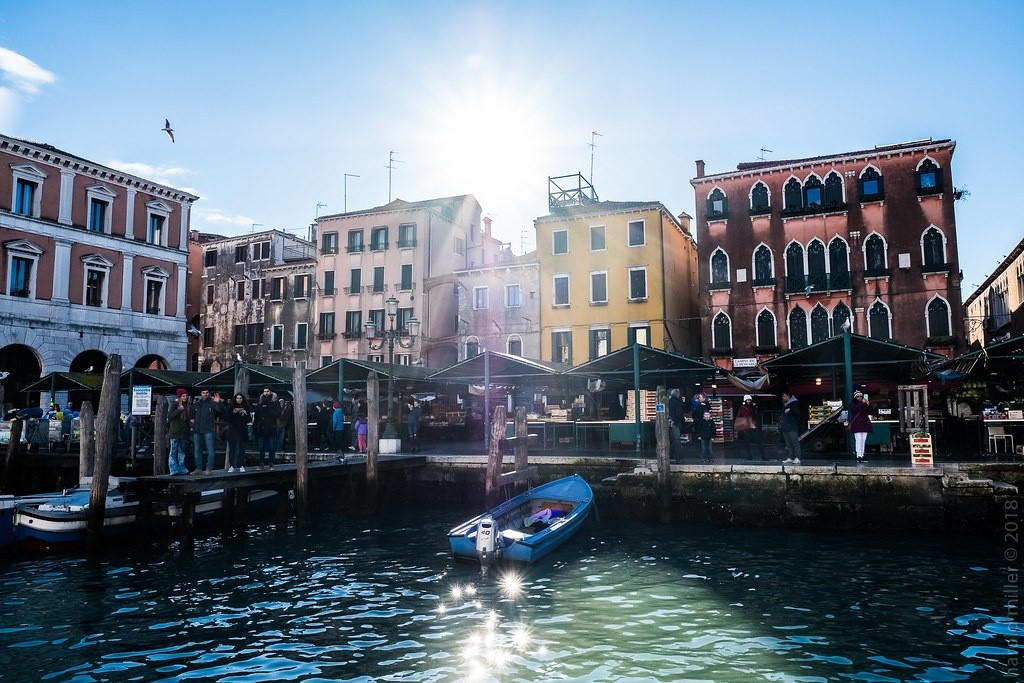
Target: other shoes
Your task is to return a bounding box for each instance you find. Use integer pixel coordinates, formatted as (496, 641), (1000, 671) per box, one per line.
(333, 452), (344, 459)
(190, 470), (203, 476)
(206, 469), (212, 476)
(269, 464), (274, 470)
(228, 467), (236, 472)
(172, 472), (181, 476)
(792, 457), (801, 464)
(782, 457), (792, 463)
(239, 466), (246, 472)
(256, 465), (263, 471)
(703, 457), (714, 463)
(857, 457), (871, 463)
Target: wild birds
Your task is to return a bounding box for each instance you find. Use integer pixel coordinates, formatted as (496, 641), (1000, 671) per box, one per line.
(161, 119), (175, 142)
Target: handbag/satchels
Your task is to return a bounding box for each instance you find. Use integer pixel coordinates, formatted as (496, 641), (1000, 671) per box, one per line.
(733, 406), (750, 431)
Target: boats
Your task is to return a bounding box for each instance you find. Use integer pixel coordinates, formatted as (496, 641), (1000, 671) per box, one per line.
(445, 474), (595, 573)
(11, 485), (141, 545)
(152, 488), (281, 530)
(0, 494), (19, 547)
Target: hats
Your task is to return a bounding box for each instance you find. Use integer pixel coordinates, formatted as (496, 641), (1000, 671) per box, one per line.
(854, 390), (863, 398)
(177, 389), (187, 398)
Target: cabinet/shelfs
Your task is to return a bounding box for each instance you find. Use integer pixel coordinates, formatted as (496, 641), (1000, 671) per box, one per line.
(505, 420), (652, 450)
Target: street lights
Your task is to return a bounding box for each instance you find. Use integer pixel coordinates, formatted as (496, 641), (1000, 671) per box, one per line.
(364, 293), (422, 453)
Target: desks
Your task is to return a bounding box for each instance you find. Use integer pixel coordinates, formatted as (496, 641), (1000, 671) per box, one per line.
(982, 420), (1023, 457)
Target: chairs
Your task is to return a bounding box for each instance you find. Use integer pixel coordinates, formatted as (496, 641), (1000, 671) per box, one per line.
(988, 426), (1014, 454)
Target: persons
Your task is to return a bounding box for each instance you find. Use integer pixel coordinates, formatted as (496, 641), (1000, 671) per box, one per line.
(189, 387), (223, 476)
(348, 396), (368, 453)
(43, 401), (80, 420)
(252, 386), (281, 471)
(690, 393), (717, 463)
(167, 388), (194, 476)
(668, 388), (685, 463)
(607, 399), (625, 448)
(407, 398), (422, 453)
(850, 389), (873, 463)
(780, 392), (802, 464)
(739, 394), (771, 460)
(224, 393), (251, 473)
(277, 398), (345, 453)
